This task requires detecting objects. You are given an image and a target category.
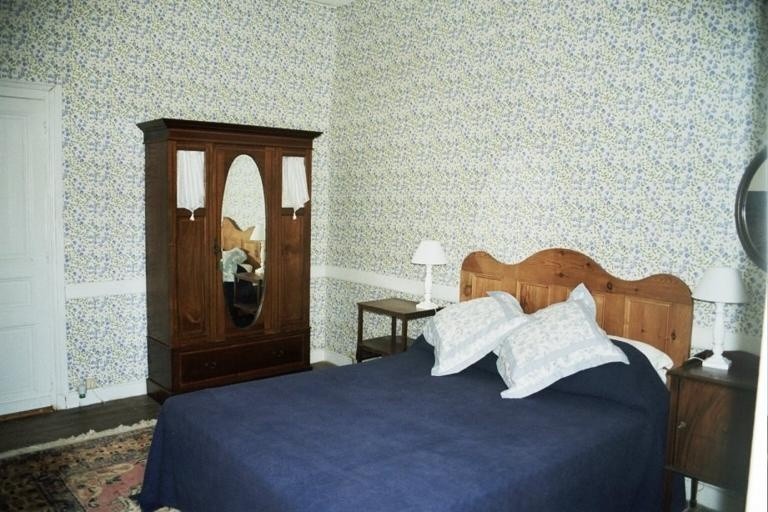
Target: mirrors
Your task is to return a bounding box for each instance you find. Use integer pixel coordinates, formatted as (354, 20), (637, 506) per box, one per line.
(219, 152), (270, 332)
(733, 143), (768, 273)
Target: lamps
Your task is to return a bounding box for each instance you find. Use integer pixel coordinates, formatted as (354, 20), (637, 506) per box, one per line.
(412, 238), (448, 310)
(249, 222), (265, 275)
(687, 260), (750, 374)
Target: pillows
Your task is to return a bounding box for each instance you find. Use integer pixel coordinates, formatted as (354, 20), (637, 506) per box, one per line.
(419, 283), (629, 400)
(221, 246), (247, 281)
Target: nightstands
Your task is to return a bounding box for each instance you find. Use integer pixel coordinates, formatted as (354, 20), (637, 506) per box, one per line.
(663, 347), (763, 512)
(232, 270), (263, 320)
(354, 296), (446, 361)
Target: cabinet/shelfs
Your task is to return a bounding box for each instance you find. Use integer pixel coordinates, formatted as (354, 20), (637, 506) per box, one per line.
(134, 117), (322, 404)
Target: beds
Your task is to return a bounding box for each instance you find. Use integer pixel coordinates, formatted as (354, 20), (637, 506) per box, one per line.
(142, 249), (694, 512)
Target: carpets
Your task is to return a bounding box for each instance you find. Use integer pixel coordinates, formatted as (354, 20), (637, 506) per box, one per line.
(0, 419), (168, 512)
